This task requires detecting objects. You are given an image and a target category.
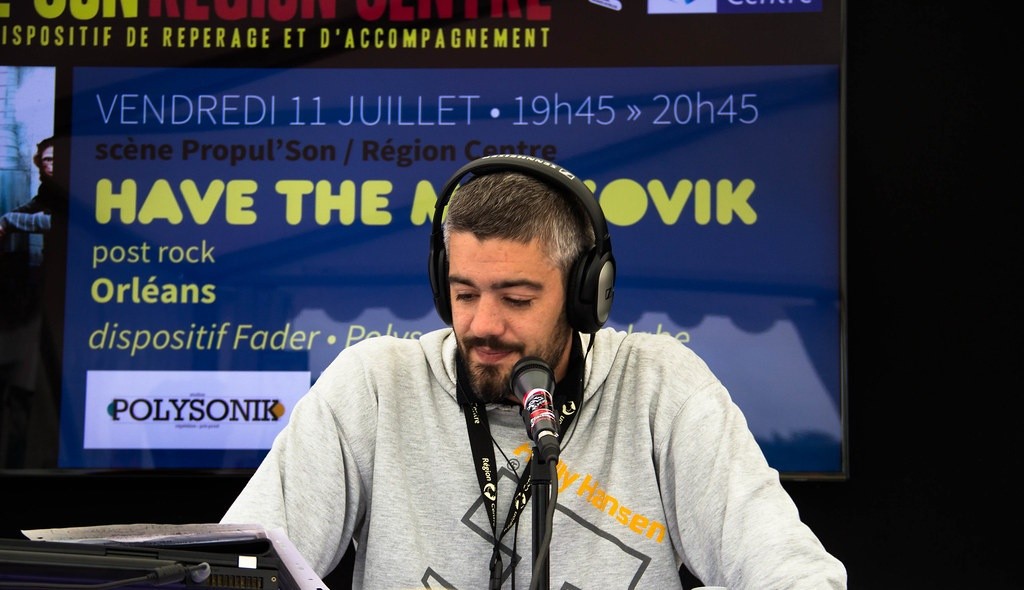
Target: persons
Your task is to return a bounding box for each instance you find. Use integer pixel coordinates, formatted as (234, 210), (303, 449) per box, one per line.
(212, 142), (861, 590)
(17, 133), (56, 215)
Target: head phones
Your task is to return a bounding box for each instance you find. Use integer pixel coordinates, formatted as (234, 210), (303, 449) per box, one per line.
(428, 152), (613, 333)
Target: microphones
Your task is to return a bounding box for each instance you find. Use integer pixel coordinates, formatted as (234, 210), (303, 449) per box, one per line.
(510, 356), (559, 462)
